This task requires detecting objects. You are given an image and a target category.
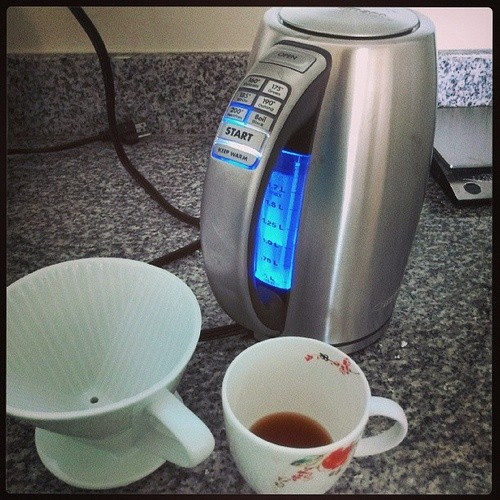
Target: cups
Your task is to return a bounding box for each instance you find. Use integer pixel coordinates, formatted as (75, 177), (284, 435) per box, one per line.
(6, 259), (217, 490)
(221, 334), (408, 496)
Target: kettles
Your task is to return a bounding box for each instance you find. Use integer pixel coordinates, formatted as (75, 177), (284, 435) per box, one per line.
(197, 4), (441, 354)
